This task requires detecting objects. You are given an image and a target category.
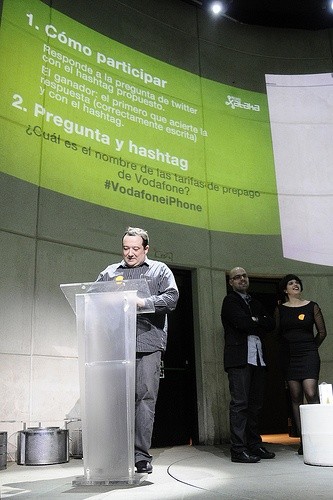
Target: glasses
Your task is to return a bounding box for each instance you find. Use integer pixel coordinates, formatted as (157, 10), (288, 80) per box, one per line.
(230, 273), (247, 280)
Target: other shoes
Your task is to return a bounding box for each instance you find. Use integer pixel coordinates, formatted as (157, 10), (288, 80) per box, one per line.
(298, 437), (303, 455)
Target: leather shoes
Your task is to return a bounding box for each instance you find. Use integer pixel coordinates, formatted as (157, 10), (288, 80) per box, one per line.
(231, 449), (261, 463)
(251, 444), (275, 459)
(136, 460), (152, 474)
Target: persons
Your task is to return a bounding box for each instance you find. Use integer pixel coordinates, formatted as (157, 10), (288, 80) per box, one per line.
(282, 274), (328, 457)
(220, 267), (276, 463)
(83, 226), (180, 474)
